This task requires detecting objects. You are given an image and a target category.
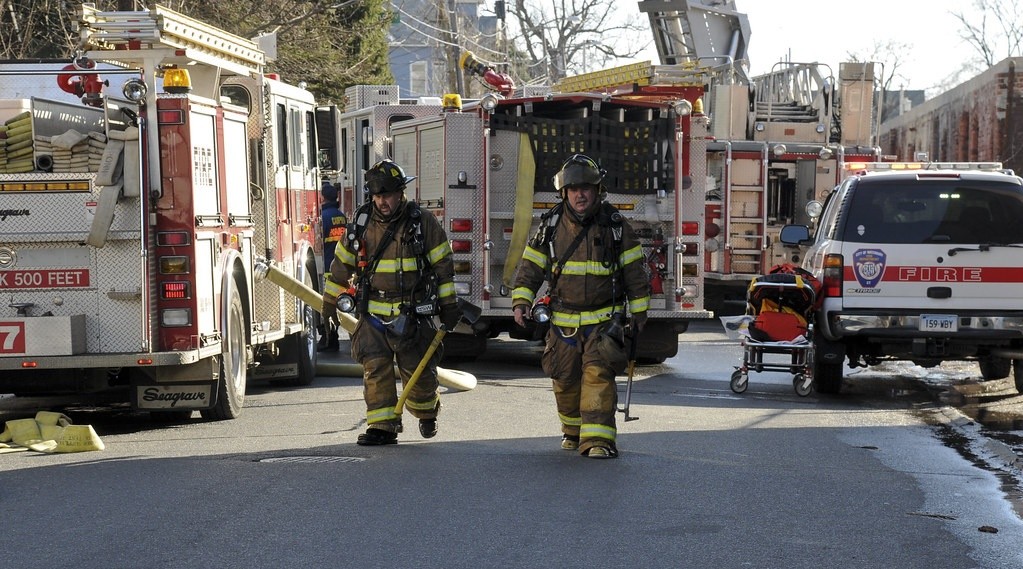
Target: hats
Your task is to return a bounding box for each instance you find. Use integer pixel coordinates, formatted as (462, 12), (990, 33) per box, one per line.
(322, 183), (338, 202)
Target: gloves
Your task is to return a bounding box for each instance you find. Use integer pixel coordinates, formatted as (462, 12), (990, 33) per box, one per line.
(440, 304), (458, 331)
(320, 301), (340, 335)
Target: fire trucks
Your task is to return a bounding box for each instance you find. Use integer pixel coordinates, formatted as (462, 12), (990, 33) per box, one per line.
(332, 43), (722, 362)
(0, 4), (345, 422)
(704, 132), (901, 321)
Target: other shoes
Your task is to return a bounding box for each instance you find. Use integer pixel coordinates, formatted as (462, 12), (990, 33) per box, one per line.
(357, 430), (399, 445)
(589, 446), (608, 458)
(317, 341), (339, 352)
(419, 417), (438, 439)
(561, 436), (578, 450)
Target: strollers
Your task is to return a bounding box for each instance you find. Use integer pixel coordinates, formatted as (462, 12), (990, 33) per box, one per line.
(729, 264), (824, 398)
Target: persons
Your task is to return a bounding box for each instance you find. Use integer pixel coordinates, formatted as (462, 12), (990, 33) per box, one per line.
(510, 153), (651, 456)
(316, 185), (347, 353)
(321, 160), (459, 447)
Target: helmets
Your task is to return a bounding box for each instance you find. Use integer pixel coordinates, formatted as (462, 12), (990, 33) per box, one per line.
(363, 158), (419, 195)
(553, 154), (607, 201)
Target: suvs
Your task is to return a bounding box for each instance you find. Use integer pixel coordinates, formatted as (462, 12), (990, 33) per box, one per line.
(779, 159), (1023, 398)
